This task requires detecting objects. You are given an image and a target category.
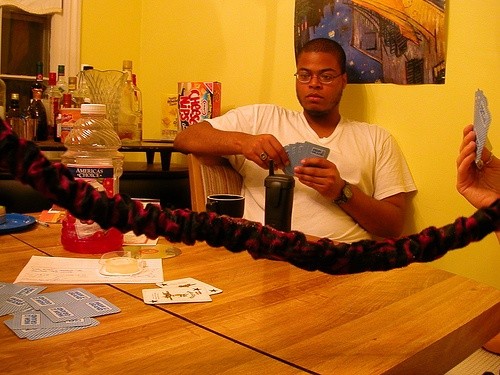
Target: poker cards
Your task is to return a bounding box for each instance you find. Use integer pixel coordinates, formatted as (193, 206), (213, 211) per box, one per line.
(281, 141), (331, 177)
(474, 89), (493, 165)
(0, 281), (122, 340)
(142, 277), (223, 305)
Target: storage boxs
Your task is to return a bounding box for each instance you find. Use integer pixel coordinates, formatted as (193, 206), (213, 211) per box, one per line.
(178, 81), (222, 131)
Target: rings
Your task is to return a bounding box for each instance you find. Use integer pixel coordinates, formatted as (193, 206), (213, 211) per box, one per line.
(261, 153), (267, 160)
(485, 153), (494, 164)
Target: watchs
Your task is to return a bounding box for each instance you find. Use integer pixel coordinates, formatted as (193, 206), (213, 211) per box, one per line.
(334, 181), (352, 206)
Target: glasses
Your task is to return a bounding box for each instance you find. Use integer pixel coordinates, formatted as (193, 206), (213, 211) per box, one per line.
(294, 71), (344, 84)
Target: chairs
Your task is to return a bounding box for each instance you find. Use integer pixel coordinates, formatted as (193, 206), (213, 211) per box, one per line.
(186, 151), (243, 214)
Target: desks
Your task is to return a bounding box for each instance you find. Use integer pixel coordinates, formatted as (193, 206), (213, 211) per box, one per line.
(0, 210), (500, 375)
(36, 135), (189, 210)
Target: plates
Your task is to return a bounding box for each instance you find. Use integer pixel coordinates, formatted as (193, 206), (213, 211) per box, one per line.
(0, 212), (37, 233)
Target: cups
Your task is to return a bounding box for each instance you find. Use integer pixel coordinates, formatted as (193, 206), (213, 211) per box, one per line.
(205, 193), (245, 218)
(8, 117), (37, 143)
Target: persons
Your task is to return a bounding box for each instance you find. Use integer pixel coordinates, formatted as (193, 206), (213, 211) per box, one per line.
(456, 125), (500, 245)
(174, 38), (418, 244)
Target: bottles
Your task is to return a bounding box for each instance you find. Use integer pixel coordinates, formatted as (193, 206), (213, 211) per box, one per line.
(60, 103), (124, 253)
(30, 62), (47, 109)
(4, 90), (22, 128)
(117, 60), (143, 148)
(43, 64), (77, 143)
(83, 65), (94, 70)
(28, 88), (48, 142)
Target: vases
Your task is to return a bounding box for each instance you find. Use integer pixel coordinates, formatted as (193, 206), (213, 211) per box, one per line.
(81, 69), (128, 137)
